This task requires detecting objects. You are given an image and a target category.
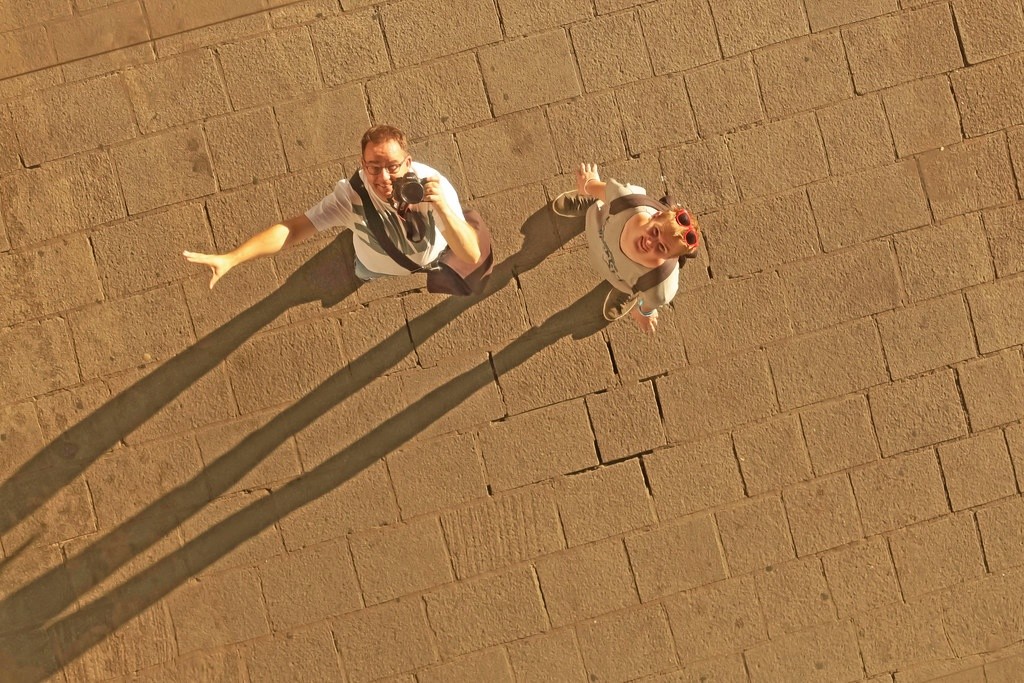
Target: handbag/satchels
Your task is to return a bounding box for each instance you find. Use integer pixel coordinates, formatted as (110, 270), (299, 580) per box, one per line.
(427, 209), (494, 295)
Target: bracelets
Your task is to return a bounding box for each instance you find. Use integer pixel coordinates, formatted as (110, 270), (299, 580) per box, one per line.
(584, 177), (598, 195)
(638, 301), (655, 317)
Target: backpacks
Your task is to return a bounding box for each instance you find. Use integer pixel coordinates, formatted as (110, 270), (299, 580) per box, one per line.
(611, 193), (697, 294)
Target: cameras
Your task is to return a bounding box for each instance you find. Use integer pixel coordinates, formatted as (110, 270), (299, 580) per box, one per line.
(393, 171), (433, 204)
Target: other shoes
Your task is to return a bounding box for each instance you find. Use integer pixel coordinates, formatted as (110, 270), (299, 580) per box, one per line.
(353, 255), (376, 287)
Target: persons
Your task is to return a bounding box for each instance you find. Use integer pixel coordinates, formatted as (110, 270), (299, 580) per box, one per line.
(183, 126), (482, 290)
(553, 163), (698, 336)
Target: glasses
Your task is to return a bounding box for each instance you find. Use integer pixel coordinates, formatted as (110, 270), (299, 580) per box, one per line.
(362, 152), (410, 175)
(677, 207), (699, 248)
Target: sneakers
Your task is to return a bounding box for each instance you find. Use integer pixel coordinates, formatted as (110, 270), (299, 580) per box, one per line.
(551, 188), (596, 218)
(603, 288), (636, 322)
